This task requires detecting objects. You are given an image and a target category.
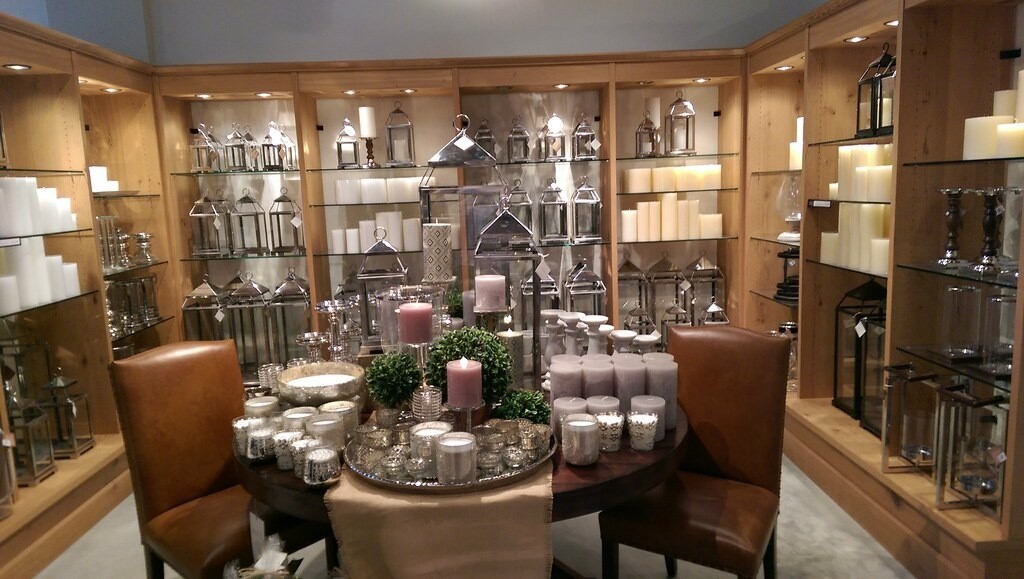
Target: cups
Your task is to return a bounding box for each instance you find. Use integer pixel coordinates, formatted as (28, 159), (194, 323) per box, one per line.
(985, 294), (1015, 361)
(287, 357), (316, 369)
(957, 436), (1003, 494)
(903, 410), (934, 465)
(231, 394), (342, 487)
(554, 396), (586, 444)
(778, 321), (798, 393)
(436, 432), (476, 484)
(549, 352), (679, 430)
(627, 410), (658, 450)
(258, 363), (284, 388)
(595, 410), (624, 451)
(561, 414), (598, 465)
(631, 395), (665, 440)
(375, 285), (444, 360)
(412, 386), (443, 422)
(318, 401), (358, 444)
(409, 421), (455, 479)
(938, 286), (981, 358)
(113, 277), (162, 326)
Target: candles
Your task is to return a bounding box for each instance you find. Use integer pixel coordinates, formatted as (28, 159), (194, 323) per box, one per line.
(422, 217), (453, 281)
(620, 162), (723, 244)
(493, 327), (526, 393)
(358, 106), (378, 138)
(789, 115), (803, 171)
(819, 142), (894, 277)
(472, 273), (506, 312)
(0, 175), (81, 316)
(546, 349), (679, 447)
(398, 298), (434, 345)
(445, 356), (483, 408)
(962, 69), (1024, 160)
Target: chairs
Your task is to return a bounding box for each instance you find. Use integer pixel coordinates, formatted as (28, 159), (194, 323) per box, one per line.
(597, 324), (792, 579)
(106, 338), (340, 579)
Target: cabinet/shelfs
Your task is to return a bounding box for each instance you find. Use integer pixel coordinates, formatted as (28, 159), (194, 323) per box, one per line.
(873, 0), (1024, 579)
(791, 1), (901, 540)
(290, 68), (465, 364)
(149, 72), (330, 397)
(67, 49), (174, 422)
(743, 26), (810, 453)
(603, 59), (745, 353)
(0, 24), (120, 579)
(455, 67), (615, 349)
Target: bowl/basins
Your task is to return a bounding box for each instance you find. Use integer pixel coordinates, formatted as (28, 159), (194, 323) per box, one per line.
(473, 418), (552, 476)
(277, 362), (366, 405)
(353, 409), (428, 484)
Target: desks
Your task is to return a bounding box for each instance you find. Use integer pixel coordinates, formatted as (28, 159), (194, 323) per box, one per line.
(225, 400), (688, 579)
